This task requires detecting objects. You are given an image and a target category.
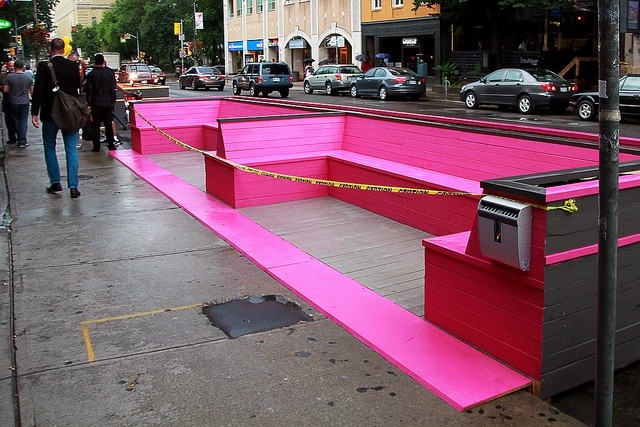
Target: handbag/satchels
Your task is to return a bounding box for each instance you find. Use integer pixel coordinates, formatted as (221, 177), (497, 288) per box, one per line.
(46, 61), (88, 130)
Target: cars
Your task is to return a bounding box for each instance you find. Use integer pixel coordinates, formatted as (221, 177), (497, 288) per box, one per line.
(460, 67), (578, 115)
(570, 74), (639, 123)
(179, 65), (226, 90)
(303, 63), (363, 95)
(349, 66), (426, 100)
(148, 66), (165, 84)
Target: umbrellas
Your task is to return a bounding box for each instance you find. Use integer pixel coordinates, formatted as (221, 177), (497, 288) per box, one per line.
(174, 64), (182, 68)
(318, 59), (334, 65)
(374, 52), (391, 59)
(355, 55), (370, 62)
(302, 58), (315, 63)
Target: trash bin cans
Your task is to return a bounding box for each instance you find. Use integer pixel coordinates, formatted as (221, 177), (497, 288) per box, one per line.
(292, 71), (299, 82)
(418, 63), (427, 77)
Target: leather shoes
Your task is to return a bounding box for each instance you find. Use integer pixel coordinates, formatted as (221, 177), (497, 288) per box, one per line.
(7, 140), (16, 144)
(91, 148), (98, 151)
(47, 183), (63, 192)
(109, 144), (117, 152)
(70, 188), (81, 198)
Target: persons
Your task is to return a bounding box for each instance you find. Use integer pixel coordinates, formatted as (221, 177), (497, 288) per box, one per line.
(101, 59), (130, 145)
(68, 51), (79, 63)
(3, 60), (34, 148)
(361, 62), (372, 73)
(86, 54), (116, 154)
(175, 66), (180, 80)
(374, 59), (387, 67)
(78, 59), (94, 96)
(0, 60), (17, 145)
(24, 65), (33, 101)
(31, 37), (81, 199)
(305, 63), (314, 78)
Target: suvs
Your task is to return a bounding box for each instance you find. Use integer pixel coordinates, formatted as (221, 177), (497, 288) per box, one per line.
(119, 63), (158, 84)
(233, 61), (293, 98)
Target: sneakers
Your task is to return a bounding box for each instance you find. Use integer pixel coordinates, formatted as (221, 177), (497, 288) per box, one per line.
(100, 136), (106, 142)
(18, 143), (30, 148)
(113, 140), (121, 146)
